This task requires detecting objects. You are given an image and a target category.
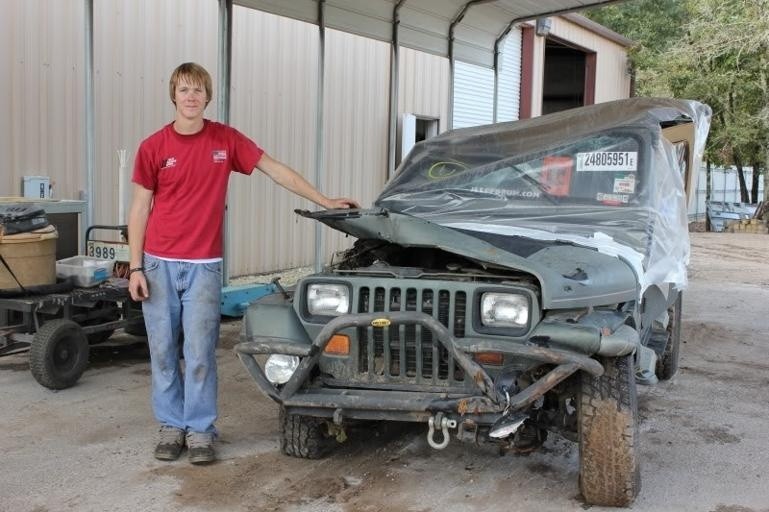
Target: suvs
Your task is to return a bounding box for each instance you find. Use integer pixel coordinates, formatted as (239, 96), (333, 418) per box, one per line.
(234, 89), (713, 506)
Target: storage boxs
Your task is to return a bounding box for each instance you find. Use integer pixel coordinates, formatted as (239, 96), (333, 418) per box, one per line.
(55, 254), (119, 291)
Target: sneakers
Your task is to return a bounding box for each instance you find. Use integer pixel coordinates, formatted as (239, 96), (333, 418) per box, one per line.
(155, 427), (214, 465)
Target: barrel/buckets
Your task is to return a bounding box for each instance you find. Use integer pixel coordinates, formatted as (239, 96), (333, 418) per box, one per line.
(0, 226), (59, 292)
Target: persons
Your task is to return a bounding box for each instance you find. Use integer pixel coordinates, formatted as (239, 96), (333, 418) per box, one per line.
(128, 62), (362, 463)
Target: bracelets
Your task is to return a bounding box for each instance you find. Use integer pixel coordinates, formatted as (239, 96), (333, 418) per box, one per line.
(129, 268), (143, 275)
